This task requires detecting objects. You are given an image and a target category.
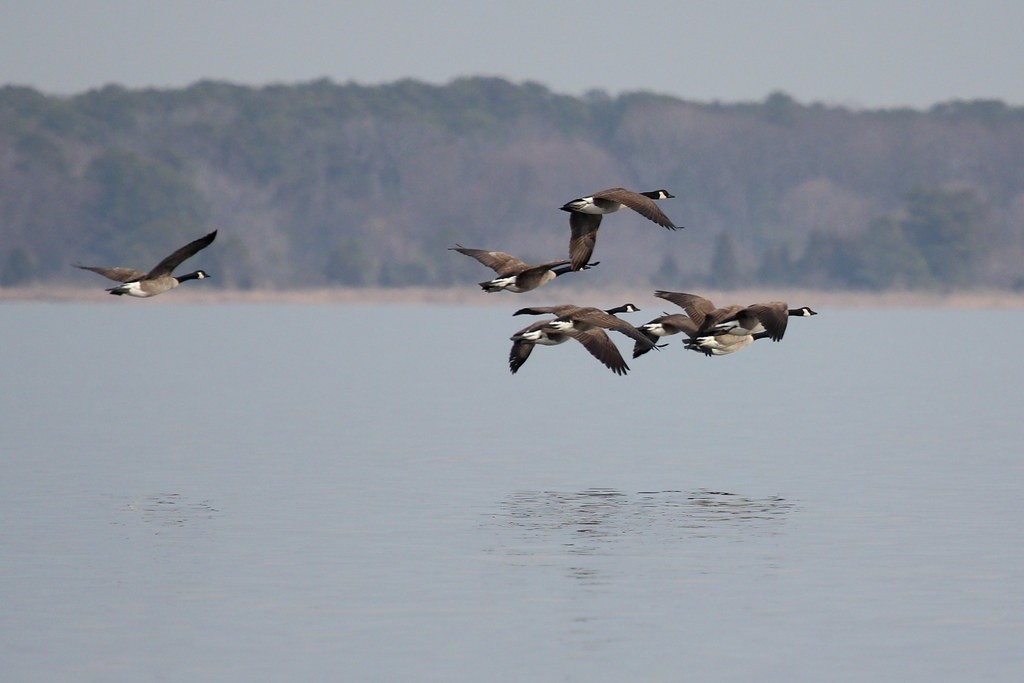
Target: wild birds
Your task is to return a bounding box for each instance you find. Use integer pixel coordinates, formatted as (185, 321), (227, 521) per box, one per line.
(66, 227), (221, 297)
(446, 187), (684, 293)
(508, 290), (819, 376)
